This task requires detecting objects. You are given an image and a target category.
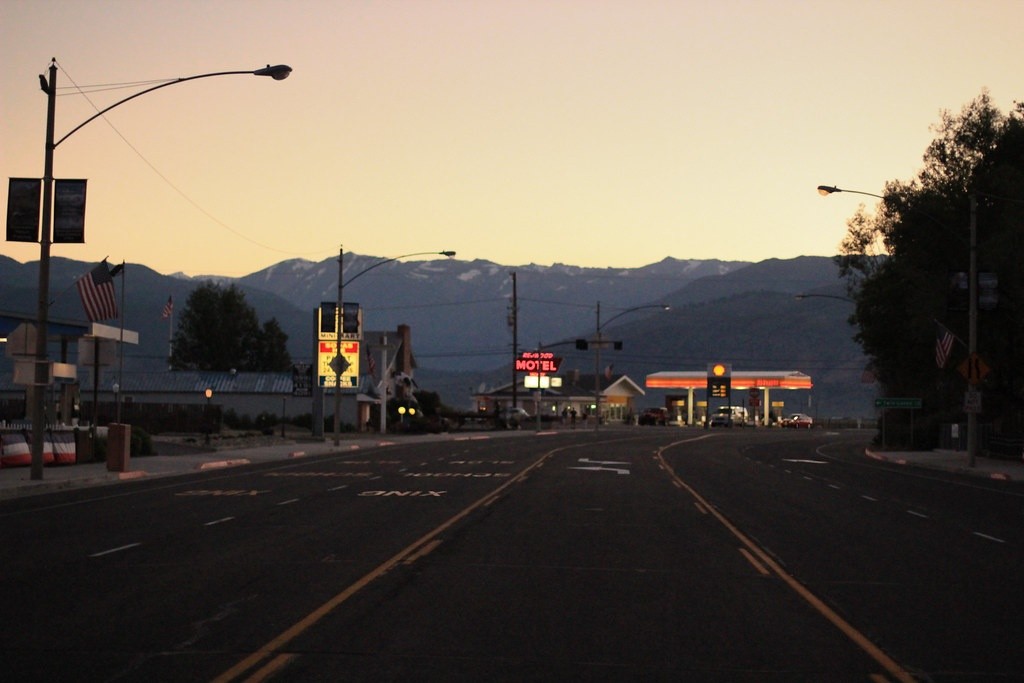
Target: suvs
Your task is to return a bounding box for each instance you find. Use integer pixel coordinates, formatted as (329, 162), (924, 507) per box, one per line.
(639, 406), (668, 427)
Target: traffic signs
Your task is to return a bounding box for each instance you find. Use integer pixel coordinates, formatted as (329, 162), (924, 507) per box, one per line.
(874, 397), (922, 409)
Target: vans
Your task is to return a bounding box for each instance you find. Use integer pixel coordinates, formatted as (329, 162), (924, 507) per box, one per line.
(709, 405), (750, 428)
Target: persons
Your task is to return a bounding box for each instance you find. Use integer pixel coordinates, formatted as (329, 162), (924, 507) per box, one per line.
(570, 408), (576, 425)
(581, 413), (588, 428)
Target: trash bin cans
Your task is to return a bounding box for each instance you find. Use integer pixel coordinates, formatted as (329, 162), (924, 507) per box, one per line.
(107, 423), (131, 472)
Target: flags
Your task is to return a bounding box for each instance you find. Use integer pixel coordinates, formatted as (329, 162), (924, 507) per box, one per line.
(605, 360), (614, 380)
(159, 297), (172, 320)
(364, 347), (379, 377)
(76, 261), (119, 323)
(935, 323), (954, 370)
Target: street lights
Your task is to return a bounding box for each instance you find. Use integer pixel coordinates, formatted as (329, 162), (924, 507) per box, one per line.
(29, 55), (294, 480)
(113, 382), (119, 421)
(595, 300), (669, 426)
(204, 386), (213, 446)
(818, 185), (980, 471)
(332, 243), (456, 447)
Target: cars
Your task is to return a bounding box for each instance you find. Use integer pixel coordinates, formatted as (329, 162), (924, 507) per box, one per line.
(781, 413), (813, 430)
(498, 407), (567, 431)
